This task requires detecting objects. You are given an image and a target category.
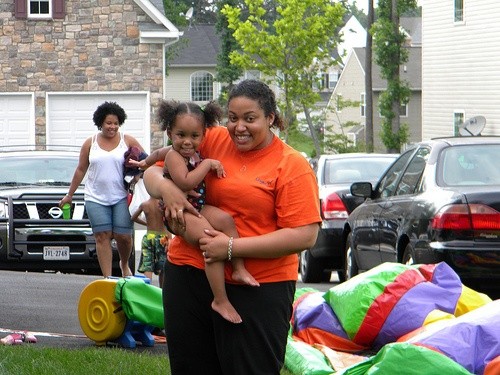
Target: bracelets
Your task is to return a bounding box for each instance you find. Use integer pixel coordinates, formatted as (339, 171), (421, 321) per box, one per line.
(66, 194), (72, 198)
(228, 237), (233, 261)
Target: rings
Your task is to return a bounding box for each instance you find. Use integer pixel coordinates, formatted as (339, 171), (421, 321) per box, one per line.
(177, 210), (183, 213)
(203, 252), (205, 257)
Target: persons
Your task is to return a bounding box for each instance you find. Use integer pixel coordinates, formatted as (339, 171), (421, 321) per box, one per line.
(128, 97), (259, 323)
(59, 102), (148, 279)
(143, 79), (322, 375)
(132, 195), (172, 285)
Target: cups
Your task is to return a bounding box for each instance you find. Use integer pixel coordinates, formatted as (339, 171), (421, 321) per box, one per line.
(62, 203), (71, 219)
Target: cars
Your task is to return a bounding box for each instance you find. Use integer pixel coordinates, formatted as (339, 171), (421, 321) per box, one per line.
(0, 153), (136, 278)
(342, 136), (500, 299)
(295, 152), (402, 282)
